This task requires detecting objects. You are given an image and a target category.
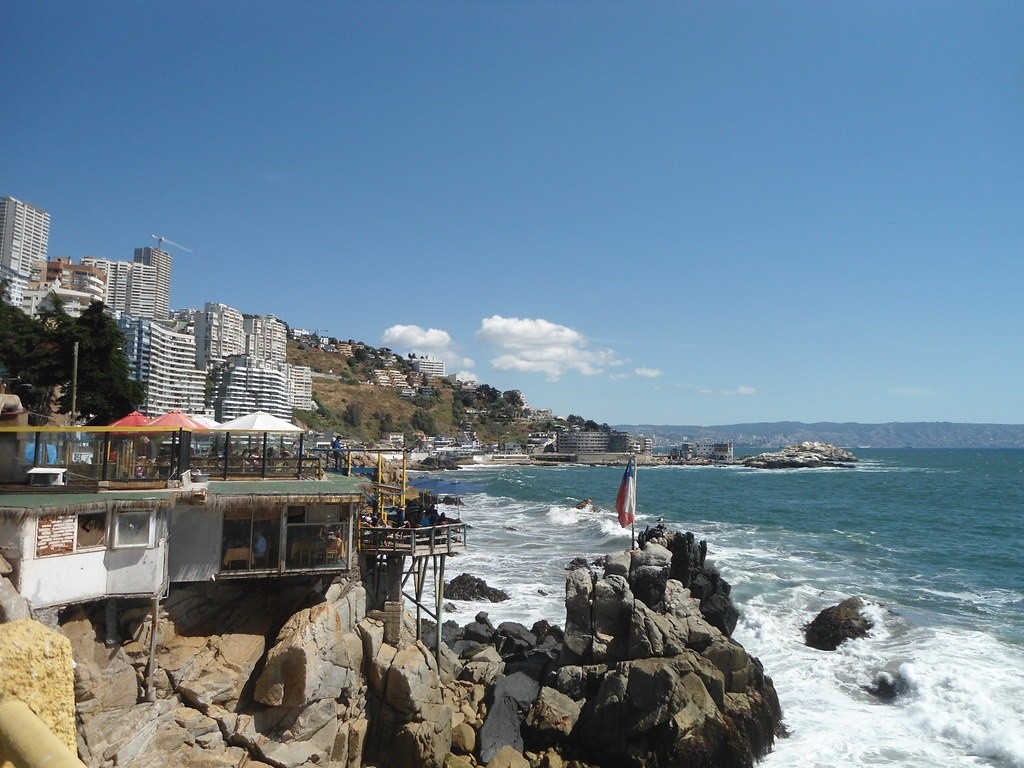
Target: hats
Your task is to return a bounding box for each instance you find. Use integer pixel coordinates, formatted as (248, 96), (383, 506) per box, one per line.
(336, 436), (342, 439)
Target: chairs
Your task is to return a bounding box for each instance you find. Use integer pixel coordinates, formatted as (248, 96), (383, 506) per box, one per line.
(290, 540), (342, 566)
(141, 457), (324, 479)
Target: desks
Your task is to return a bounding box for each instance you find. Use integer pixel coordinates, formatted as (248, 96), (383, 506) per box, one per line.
(223, 548), (255, 569)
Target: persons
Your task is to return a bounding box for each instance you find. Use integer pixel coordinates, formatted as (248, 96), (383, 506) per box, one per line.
(364, 498), (449, 546)
(332, 435), (348, 471)
(186, 440), (302, 473)
(251, 529), (267, 567)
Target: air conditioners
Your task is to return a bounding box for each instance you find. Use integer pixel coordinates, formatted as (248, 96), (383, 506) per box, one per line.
(31, 473), (63, 486)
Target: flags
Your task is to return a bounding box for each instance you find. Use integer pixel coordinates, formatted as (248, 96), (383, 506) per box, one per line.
(614, 458), (638, 527)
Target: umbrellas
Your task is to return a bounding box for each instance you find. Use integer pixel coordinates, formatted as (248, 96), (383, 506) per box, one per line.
(92, 408), (219, 438)
(213, 411), (308, 436)
